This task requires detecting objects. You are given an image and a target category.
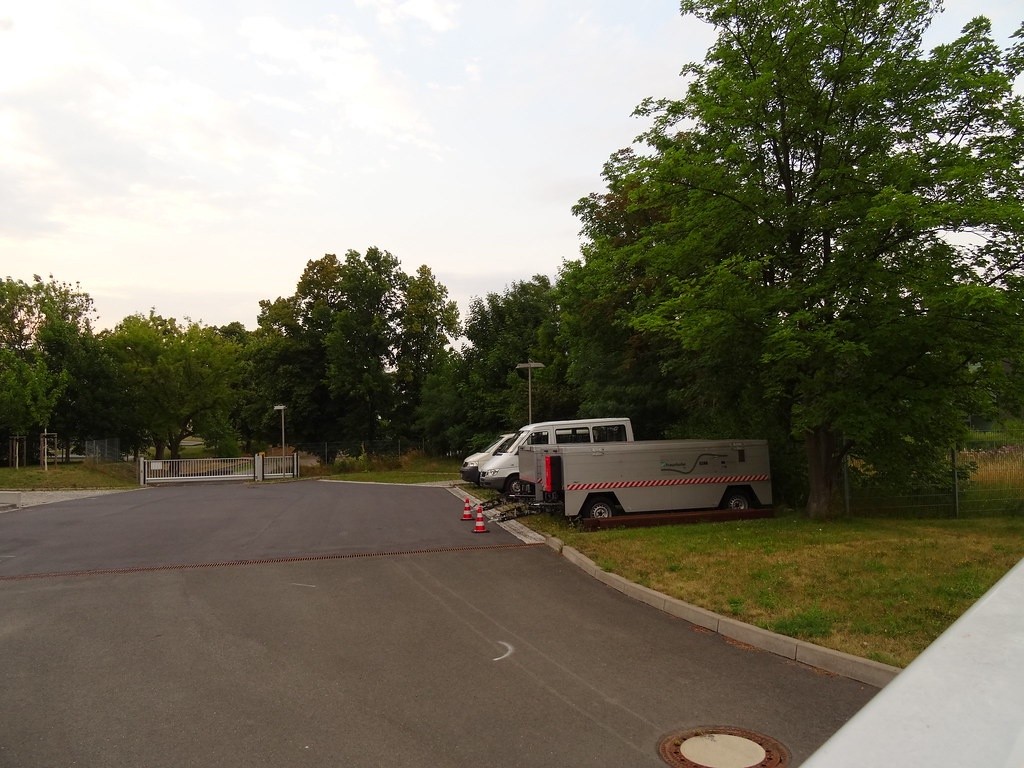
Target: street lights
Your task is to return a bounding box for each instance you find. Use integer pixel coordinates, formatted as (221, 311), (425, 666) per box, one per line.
(273, 404), (287, 480)
(516, 362), (549, 426)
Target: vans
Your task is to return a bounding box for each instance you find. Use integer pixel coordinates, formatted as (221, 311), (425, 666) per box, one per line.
(459, 432), (517, 483)
(478, 417), (635, 492)
